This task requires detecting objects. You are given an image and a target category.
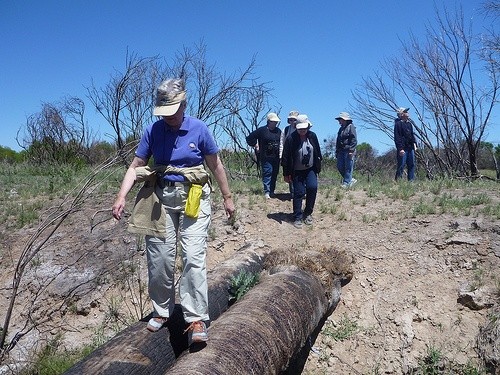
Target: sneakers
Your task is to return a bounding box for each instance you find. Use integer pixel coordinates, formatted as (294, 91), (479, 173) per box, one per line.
(340, 184), (347, 188)
(147, 317), (170, 331)
(188, 321), (208, 343)
(265, 194), (270, 199)
(349, 178), (357, 190)
(304, 214), (313, 225)
(293, 220), (303, 229)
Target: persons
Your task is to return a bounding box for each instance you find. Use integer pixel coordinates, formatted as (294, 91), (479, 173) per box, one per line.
(279, 111), (306, 200)
(334, 111), (357, 188)
(246, 112), (282, 200)
(282, 114), (322, 229)
(112, 78), (236, 343)
(394, 107), (417, 183)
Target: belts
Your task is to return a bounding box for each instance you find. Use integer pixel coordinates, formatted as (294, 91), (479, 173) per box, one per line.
(162, 181), (189, 186)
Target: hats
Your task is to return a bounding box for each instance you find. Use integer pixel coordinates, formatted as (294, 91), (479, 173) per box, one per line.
(296, 115), (313, 129)
(153, 78), (186, 116)
(397, 108), (411, 117)
(335, 112), (353, 121)
(287, 111), (299, 120)
(267, 112), (281, 121)
